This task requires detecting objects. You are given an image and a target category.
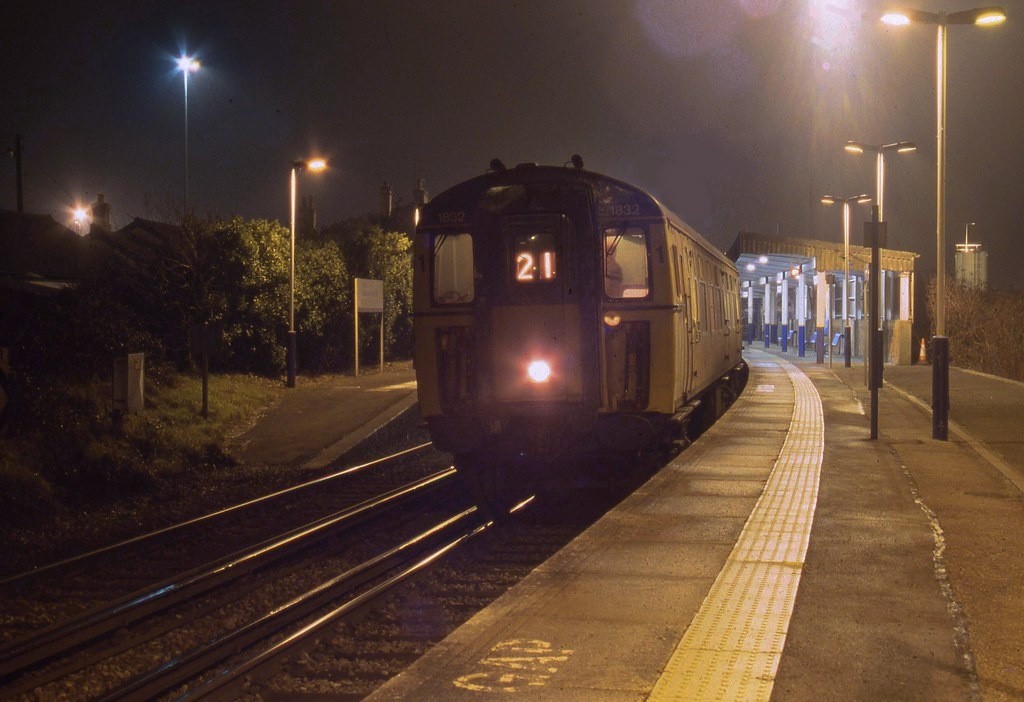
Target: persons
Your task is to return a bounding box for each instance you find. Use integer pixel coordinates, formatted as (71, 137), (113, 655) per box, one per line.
(606, 243), (622, 297)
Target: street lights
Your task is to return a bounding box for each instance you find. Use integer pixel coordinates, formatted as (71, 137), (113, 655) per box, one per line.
(288, 158), (329, 386)
(821, 193), (870, 366)
(878, 6), (1010, 441)
(845, 139), (918, 387)
(177, 59), (209, 415)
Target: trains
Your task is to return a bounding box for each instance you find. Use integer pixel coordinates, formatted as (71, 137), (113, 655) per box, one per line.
(412, 156), (743, 523)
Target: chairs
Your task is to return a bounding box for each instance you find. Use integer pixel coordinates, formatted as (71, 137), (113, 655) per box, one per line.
(777, 329), (797, 347)
(805, 331), (817, 352)
(824, 333), (844, 354)
